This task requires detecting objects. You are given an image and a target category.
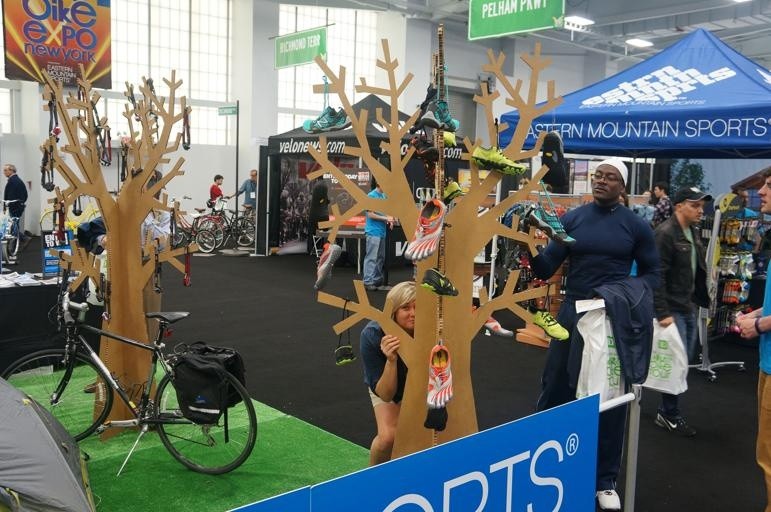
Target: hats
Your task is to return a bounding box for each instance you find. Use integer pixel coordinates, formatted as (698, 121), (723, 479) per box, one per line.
(596, 159), (629, 188)
(675, 185), (712, 203)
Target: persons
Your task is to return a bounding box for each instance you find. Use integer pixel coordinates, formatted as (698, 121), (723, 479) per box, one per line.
(734, 169), (771, 512)
(3, 164), (32, 253)
(210, 175), (228, 236)
(618, 181), (673, 230)
(727, 186), (763, 251)
(77, 172), (172, 394)
(360, 280), (416, 467)
(528, 158), (662, 512)
(362, 180), (394, 291)
(228, 169), (257, 231)
(636, 185), (712, 438)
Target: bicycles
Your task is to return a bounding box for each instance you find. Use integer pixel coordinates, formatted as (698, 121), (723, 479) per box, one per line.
(0, 197), (23, 255)
(1, 281), (255, 477)
(171, 195), (255, 252)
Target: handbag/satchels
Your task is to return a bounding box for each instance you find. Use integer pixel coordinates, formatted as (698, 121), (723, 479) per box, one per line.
(173, 341), (246, 424)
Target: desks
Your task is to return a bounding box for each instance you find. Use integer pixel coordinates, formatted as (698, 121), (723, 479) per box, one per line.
(310, 230), (366, 274)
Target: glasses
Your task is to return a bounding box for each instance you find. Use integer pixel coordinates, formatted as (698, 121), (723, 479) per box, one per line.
(591, 173), (623, 186)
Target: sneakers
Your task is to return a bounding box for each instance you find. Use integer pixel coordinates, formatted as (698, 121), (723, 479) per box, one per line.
(406, 99), (460, 187)
(335, 344), (357, 366)
(404, 199), (446, 261)
(655, 413), (696, 437)
(470, 146), (526, 176)
(529, 207), (576, 245)
(364, 285), (393, 291)
(302, 107), (352, 134)
(420, 268), (458, 296)
(472, 306), (514, 337)
(541, 131), (564, 169)
(314, 242), (342, 289)
(434, 177), (462, 205)
(427, 345), (454, 409)
(597, 490), (622, 510)
(532, 311), (569, 341)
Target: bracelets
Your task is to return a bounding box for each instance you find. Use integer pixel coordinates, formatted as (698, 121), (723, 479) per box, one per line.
(755, 318), (765, 334)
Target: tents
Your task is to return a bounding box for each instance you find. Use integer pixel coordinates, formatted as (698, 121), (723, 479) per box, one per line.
(0, 366), (95, 512)
